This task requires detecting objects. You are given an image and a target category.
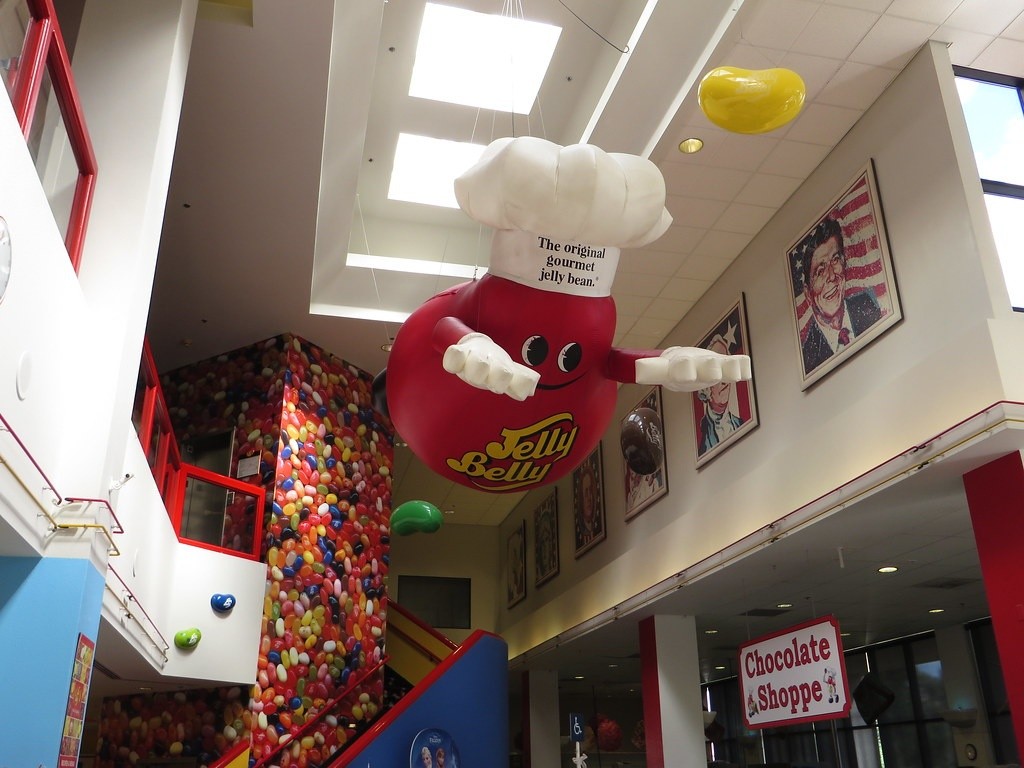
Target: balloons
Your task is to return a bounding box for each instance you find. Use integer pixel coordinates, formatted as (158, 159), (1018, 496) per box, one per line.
(621, 406), (665, 475)
(390, 500), (443, 537)
(698, 66), (806, 134)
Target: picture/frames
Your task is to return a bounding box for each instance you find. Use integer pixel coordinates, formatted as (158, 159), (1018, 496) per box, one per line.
(616, 386), (669, 522)
(568, 440), (606, 559)
(531, 485), (560, 588)
(783, 159), (906, 391)
(505, 518), (527, 610)
(690, 292), (759, 470)
